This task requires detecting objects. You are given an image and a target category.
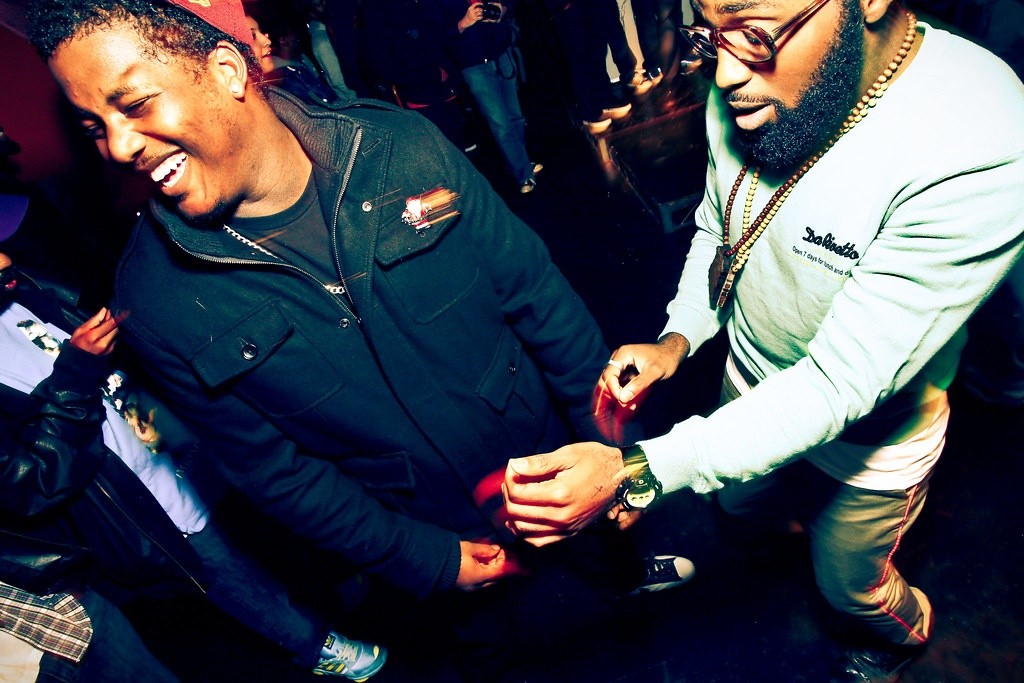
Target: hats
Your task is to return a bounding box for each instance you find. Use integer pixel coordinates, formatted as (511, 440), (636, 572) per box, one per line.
(2, 2), (259, 49)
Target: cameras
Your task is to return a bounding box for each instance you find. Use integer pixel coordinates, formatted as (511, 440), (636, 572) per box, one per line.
(475, 3), (502, 20)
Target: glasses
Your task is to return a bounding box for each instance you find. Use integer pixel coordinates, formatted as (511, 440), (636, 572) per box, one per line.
(678, 0), (829, 65)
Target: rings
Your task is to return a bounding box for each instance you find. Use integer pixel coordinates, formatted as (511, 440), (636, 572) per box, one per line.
(604, 360), (623, 369)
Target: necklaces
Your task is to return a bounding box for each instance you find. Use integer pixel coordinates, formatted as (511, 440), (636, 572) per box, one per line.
(223, 223), (348, 295)
(707, 9), (916, 305)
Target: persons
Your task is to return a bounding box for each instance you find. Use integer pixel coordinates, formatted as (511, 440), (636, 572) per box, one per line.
(501, 1), (1024, 683)
(27, 1), (693, 683)
(243, 0), (702, 193)
(0, 128), (388, 683)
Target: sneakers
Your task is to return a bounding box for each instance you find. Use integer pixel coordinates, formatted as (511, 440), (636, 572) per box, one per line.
(518, 178), (535, 194)
(628, 553), (694, 599)
(577, 103), (612, 126)
(836, 582), (933, 683)
(528, 161), (543, 174)
(316, 632), (386, 681)
(599, 93), (632, 115)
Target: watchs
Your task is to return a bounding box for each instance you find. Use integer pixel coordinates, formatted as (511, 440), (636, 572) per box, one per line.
(616, 445), (662, 511)
